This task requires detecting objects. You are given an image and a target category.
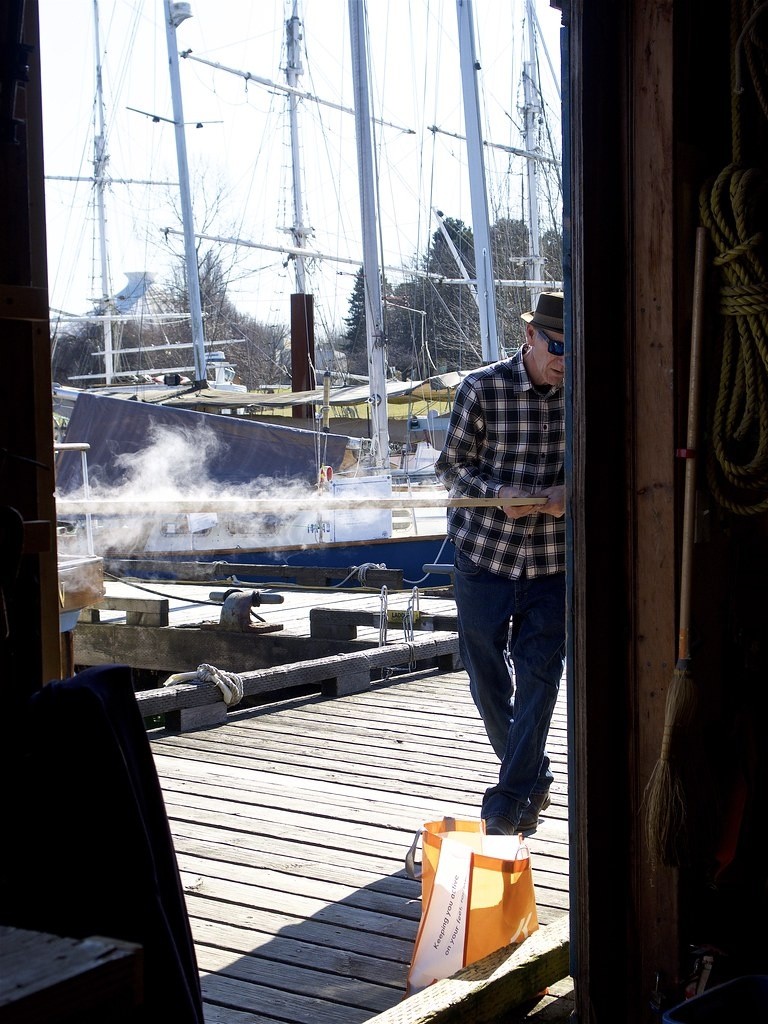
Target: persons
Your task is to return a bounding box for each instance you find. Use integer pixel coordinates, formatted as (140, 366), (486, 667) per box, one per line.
(432, 292), (566, 836)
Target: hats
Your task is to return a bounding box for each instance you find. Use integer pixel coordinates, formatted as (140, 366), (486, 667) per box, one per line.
(520, 292), (564, 334)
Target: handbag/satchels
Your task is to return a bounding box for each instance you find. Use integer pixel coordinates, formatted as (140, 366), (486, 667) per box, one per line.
(401, 815), (549, 1006)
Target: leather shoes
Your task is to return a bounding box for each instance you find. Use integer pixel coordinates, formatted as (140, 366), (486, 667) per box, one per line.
(484, 817), (515, 835)
(517, 792), (550, 830)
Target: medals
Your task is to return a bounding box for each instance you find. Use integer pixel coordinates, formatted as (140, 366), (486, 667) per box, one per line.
(106, 366), (481, 418)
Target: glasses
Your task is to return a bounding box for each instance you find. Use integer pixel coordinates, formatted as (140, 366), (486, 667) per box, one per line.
(537, 329), (564, 356)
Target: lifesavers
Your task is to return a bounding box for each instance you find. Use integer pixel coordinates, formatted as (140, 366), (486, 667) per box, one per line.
(316, 464), (334, 484)
(153, 373), (191, 385)
